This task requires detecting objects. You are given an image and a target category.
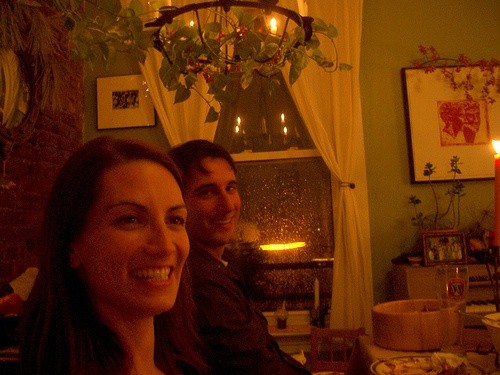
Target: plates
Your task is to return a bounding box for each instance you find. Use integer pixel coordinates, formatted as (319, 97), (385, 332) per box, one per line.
(370, 352), (485, 375)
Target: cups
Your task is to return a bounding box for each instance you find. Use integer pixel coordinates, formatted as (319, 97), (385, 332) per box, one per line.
(276, 303), (288, 328)
(436, 264), (469, 315)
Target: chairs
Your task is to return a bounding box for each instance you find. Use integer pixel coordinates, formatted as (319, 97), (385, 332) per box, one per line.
(309, 326), (365, 375)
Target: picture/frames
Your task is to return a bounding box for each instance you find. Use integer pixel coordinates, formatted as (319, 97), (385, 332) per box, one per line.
(422, 231), (470, 267)
(96, 74), (157, 130)
(400, 63), (500, 183)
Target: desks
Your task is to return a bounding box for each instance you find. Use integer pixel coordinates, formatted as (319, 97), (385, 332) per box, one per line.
(269, 322), (330, 337)
(393, 256), (500, 308)
(343, 308), (497, 375)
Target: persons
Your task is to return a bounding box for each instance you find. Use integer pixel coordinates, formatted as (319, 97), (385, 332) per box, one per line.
(167, 139), (313, 375)
(17, 133), (215, 375)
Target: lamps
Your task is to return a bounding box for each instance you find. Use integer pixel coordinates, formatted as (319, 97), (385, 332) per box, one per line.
(144, 0), (354, 89)
(232, 75), (303, 154)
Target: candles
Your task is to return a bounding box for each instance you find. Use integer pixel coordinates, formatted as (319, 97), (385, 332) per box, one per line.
(314, 279), (319, 307)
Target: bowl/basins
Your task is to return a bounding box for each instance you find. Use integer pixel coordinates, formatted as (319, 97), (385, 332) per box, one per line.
(481, 311), (500, 355)
